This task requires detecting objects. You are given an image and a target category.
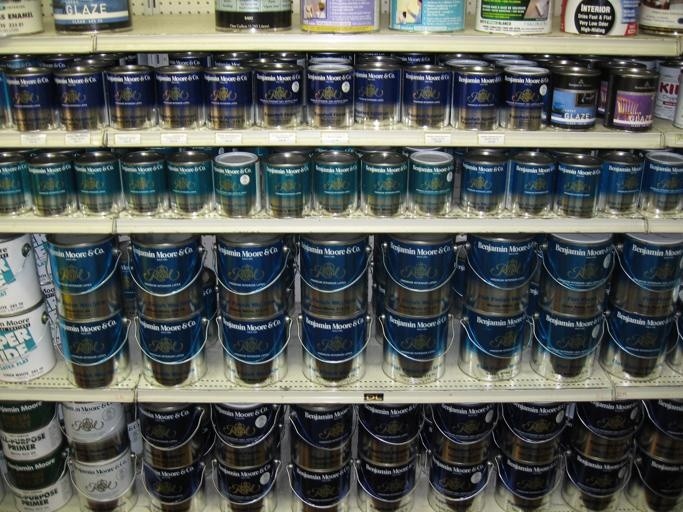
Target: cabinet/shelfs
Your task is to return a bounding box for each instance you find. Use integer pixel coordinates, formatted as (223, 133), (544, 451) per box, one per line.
(1, 13), (683, 511)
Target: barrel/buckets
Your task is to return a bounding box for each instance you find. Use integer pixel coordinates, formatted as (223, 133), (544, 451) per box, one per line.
(294, 233), (374, 320)
(37, 295), (59, 350)
(0, 400), (63, 461)
(463, 233), (542, 316)
(212, 314), (293, 388)
(285, 462), (359, 511)
(458, 312), (533, 379)
(376, 310), (455, 387)
(559, 447), (634, 512)
(0, 457), (74, 511)
(206, 402), (284, 467)
(530, 314), (606, 383)
(0, 232), (44, 317)
(286, 404), (359, 471)
(124, 235), (209, 317)
(135, 402), (206, 470)
(356, 404), (422, 467)
(499, 402), (569, 464)
(634, 450), (683, 512)
(491, 453), (570, 512)
(665, 311), (683, 375)
(139, 462), (207, 512)
(615, 232), (683, 319)
(538, 233), (618, 318)
(58, 400), (131, 464)
(595, 306), (679, 384)
(353, 457), (422, 511)
(207, 459), (283, 511)
(132, 310), (210, 388)
(30, 233), (55, 298)
(570, 402), (639, 461)
(379, 234), (462, 316)
(295, 316), (374, 387)
(0, 297), (57, 381)
(427, 404), (501, 464)
(636, 398), (683, 462)
(63, 448), (141, 512)
(210, 233), (300, 322)
(424, 459), (499, 512)
